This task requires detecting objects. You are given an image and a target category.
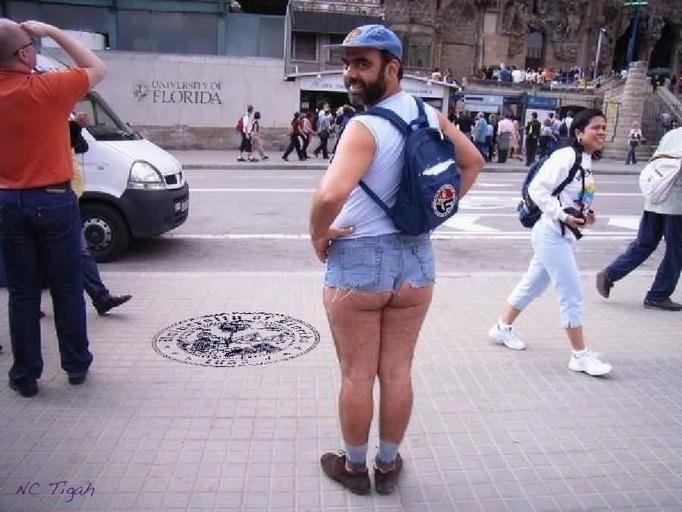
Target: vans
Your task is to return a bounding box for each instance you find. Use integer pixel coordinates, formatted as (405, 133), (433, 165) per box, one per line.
(31, 54), (190, 257)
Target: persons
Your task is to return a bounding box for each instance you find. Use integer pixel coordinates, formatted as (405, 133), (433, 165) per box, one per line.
(310, 24), (487, 494)
(40, 112), (133, 317)
(236, 63), (682, 166)
(0, 16), (107, 397)
(596, 127), (682, 311)
(489, 107), (611, 377)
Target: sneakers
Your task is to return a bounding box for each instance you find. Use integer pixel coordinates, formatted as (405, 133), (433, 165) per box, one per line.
(282, 149), (331, 161)
(489, 322), (527, 350)
(374, 454), (403, 495)
(236, 155), (270, 162)
(569, 350), (614, 377)
(644, 291), (682, 311)
(320, 451), (371, 496)
(597, 270), (614, 299)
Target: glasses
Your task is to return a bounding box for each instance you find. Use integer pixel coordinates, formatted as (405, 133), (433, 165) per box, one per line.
(13, 37), (38, 55)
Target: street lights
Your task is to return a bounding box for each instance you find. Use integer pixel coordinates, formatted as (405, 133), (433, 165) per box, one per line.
(594, 27), (610, 78)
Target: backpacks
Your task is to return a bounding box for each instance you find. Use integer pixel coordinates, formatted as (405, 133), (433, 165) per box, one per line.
(236, 116), (243, 131)
(559, 119), (567, 137)
(288, 120), (298, 135)
(639, 150), (682, 204)
(358, 95), (461, 235)
(518, 142), (582, 240)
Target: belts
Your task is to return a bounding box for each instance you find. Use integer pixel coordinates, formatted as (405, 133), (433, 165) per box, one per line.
(33, 179), (72, 189)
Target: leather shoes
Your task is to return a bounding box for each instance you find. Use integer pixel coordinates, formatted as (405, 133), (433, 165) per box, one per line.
(7, 374), (37, 397)
(67, 371), (88, 384)
(96, 295), (132, 314)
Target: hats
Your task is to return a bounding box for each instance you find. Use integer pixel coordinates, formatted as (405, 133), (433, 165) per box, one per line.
(322, 24), (403, 61)
